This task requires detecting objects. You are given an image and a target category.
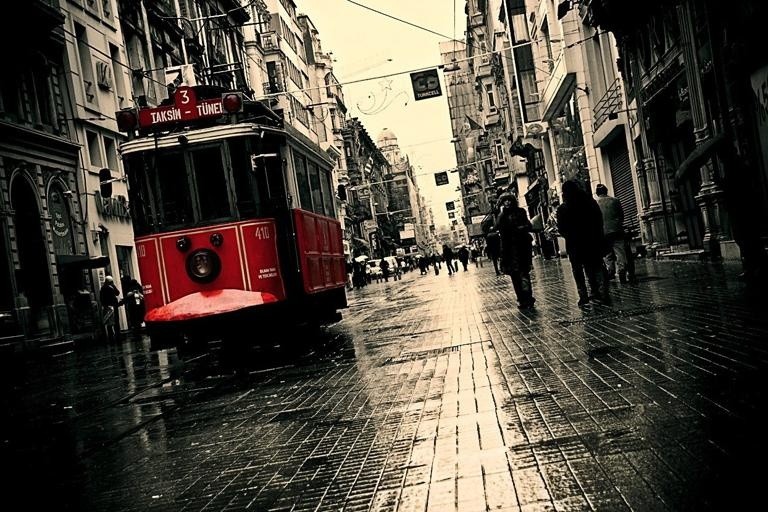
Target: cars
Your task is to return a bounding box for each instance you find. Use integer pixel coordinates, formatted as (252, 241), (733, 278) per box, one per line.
(362, 243), (471, 279)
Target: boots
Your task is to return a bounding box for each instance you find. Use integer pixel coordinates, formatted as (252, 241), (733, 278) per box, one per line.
(577, 288), (589, 306)
(588, 286), (600, 299)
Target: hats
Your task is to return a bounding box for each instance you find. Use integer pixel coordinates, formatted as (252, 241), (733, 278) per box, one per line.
(595, 183), (608, 196)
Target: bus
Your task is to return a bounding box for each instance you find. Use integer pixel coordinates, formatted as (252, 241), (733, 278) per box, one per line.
(97, 68), (354, 359)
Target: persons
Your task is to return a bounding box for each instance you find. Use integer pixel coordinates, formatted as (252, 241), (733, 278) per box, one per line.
(594, 183), (630, 286)
(556, 179), (612, 308)
(128, 278), (144, 332)
(342, 245), (479, 292)
(495, 192), (536, 310)
(101, 275), (121, 339)
(487, 226), (503, 278)
